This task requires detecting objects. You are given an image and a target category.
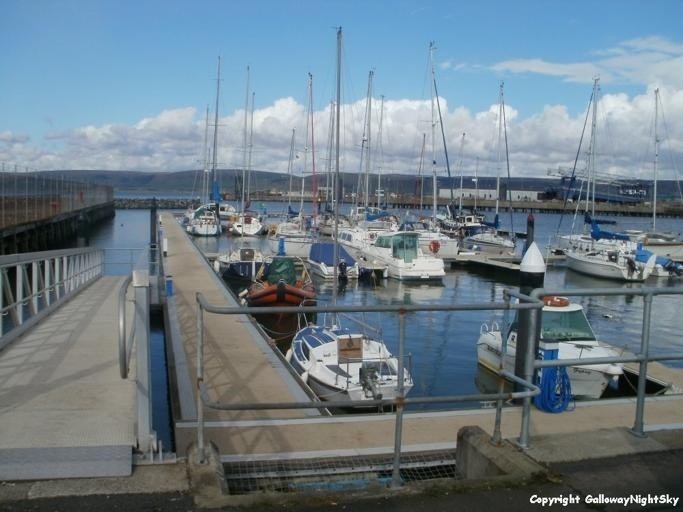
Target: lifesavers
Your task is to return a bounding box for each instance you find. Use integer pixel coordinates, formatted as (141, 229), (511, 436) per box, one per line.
(543, 294), (569, 306)
(429, 240), (441, 253)
